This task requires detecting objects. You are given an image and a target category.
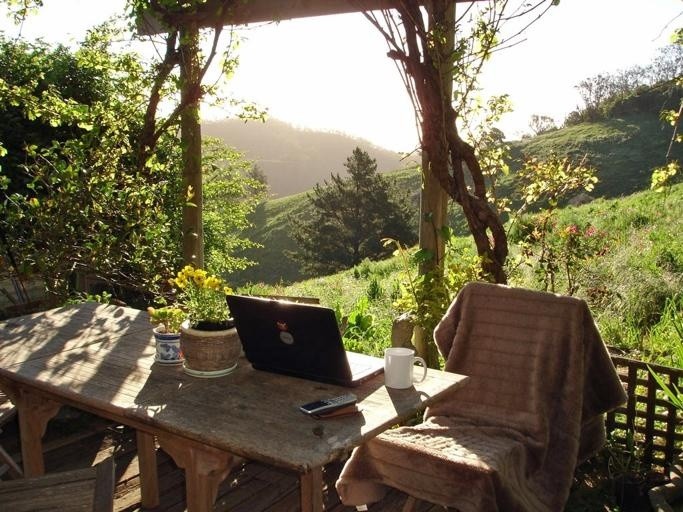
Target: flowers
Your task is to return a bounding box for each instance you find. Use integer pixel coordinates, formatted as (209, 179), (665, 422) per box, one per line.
(146, 303), (187, 333)
(168, 265), (235, 327)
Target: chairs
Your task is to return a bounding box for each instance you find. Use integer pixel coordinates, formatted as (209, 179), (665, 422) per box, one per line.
(363, 284), (588, 511)
(0, 457), (116, 512)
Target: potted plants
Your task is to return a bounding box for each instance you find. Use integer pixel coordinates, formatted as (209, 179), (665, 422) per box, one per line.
(605, 431), (651, 512)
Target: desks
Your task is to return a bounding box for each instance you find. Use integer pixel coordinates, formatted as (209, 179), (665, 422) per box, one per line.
(1, 302), (469, 511)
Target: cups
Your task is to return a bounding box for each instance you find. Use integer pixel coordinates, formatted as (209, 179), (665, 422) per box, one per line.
(383, 346), (428, 390)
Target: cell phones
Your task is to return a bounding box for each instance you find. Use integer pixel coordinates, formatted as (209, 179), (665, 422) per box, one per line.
(298, 390), (358, 416)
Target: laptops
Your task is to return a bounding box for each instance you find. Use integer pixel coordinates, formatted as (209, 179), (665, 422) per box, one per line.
(225, 295), (386, 387)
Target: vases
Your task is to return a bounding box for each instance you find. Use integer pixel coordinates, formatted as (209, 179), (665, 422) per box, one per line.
(152, 325), (185, 366)
(180, 319), (242, 378)
